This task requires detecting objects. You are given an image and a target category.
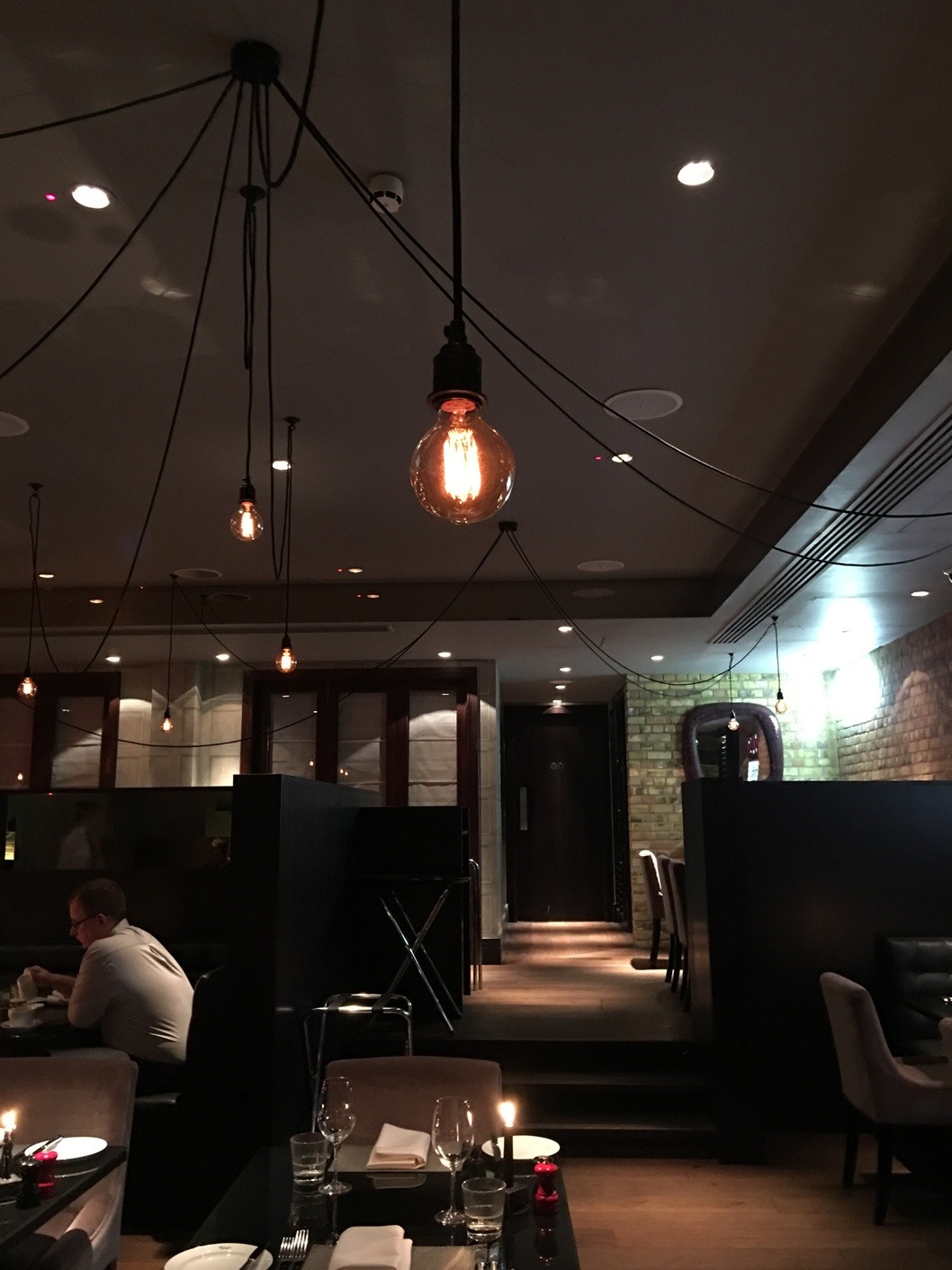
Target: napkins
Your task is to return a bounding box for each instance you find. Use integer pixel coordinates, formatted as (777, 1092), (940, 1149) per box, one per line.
(366, 1122), (431, 1171)
(327, 1224), (415, 1270)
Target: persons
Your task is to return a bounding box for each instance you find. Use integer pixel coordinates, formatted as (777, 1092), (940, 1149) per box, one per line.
(26, 878), (194, 1100)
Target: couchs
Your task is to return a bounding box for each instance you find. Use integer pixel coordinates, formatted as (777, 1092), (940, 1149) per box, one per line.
(874, 933), (952, 1062)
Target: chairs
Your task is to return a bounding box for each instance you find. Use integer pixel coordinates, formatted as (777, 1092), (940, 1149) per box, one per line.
(633, 847), (692, 1006)
(0, 1052), (138, 1270)
(134, 963), (233, 1140)
(815, 968), (952, 1231)
(323, 1054), (503, 1149)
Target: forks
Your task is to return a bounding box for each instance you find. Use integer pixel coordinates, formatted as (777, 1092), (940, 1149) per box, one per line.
(276, 1237), (294, 1270)
(295, 1230), (308, 1270)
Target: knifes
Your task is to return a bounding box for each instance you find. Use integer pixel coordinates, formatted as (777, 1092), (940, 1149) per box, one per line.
(239, 1240), (273, 1270)
(29, 1135), (63, 1160)
(489, 1242), (500, 1270)
(475, 1242), (487, 1270)
(490, 1132), (501, 1162)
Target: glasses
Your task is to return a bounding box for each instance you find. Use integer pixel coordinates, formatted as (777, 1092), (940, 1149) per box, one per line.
(70, 912), (107, 929)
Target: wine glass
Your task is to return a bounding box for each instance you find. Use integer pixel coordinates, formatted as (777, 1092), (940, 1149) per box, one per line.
(431, 1095), (475, 1225)
(317, 1077), (358, 1195)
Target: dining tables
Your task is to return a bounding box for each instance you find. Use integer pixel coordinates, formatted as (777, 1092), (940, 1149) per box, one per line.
(0, 985), (87, 1055)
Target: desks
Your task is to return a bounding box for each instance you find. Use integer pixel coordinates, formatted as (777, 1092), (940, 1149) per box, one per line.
(184, 1139), (584, 1270)
(360, 870), (473, 1035)
(900, 994), (952, 1021)
(0, 1145), (128, 1256)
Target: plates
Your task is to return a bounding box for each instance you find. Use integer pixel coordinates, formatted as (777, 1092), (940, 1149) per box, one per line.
(1, 1019), (43, 1028)
(481, 1134), (561, 1163)
(19, 1156), (108, 1178)
(27, 1003), (46, 1012)
(25, 1136), (108, 1163)
(163, 1243), (273, 1270)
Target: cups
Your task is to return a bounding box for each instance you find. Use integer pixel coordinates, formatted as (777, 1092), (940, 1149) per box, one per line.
(290, 1133), (330, 1185)
(289, 1187), (327, 1234)
(8, 1007), (34, 1026)
(462, 1176), (507, 1242)
(10, 999), (25, 1007)
